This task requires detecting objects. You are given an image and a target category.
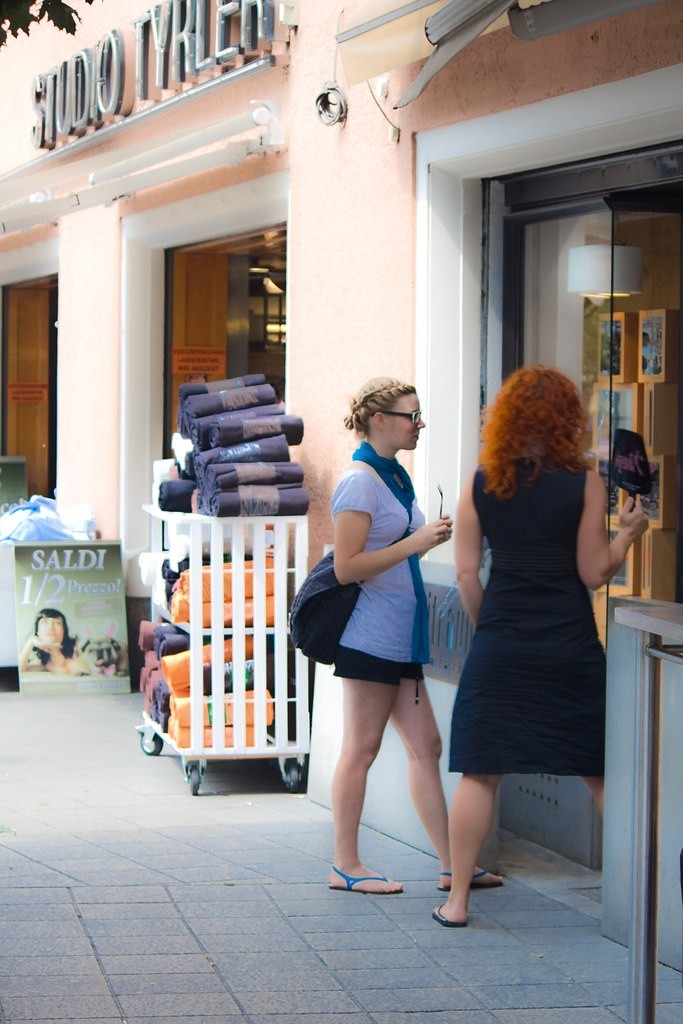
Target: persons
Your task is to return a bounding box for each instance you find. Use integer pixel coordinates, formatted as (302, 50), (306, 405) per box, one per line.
(434, 367), (649, 929)
(330, 378), (504, 894)
(19, 608), (82, 674)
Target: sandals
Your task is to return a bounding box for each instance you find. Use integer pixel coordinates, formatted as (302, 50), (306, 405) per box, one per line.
(437, 870), (504, 891)
(329, 865), (404, 894)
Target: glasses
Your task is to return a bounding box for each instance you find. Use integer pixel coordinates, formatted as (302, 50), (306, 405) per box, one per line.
(371, 410), (422, 423)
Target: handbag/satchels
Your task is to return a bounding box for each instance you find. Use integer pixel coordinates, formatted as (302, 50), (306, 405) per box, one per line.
(288, 545), (362, 665)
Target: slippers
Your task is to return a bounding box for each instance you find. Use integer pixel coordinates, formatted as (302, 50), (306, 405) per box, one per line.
(432, 903), (467, 928)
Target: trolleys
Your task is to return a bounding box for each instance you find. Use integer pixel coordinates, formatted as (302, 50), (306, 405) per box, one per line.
(135, 459), (316, 795)
